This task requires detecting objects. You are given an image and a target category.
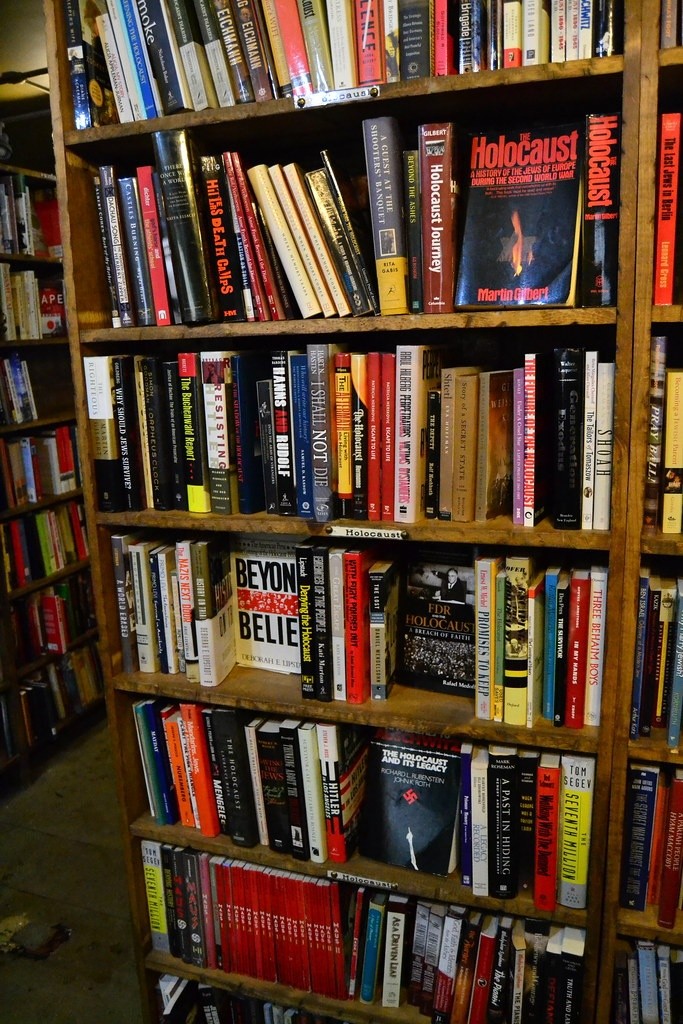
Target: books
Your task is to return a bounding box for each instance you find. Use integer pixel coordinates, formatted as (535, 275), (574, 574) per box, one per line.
(0, 1), (683, 1024)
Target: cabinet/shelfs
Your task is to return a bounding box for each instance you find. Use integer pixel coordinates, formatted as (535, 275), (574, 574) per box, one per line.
(0, 0), (683, 1024)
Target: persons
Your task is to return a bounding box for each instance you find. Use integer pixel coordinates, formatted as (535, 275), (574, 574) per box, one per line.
(434, 568), (467, 603)
